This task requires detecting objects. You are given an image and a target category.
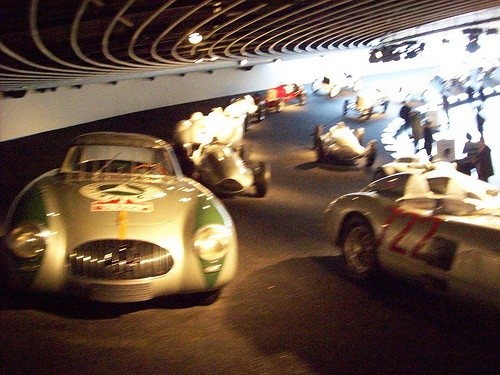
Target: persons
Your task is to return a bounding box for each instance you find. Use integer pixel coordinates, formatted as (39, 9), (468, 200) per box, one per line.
(400, 87), (495, 183)
(426, 177), (450, 194)
